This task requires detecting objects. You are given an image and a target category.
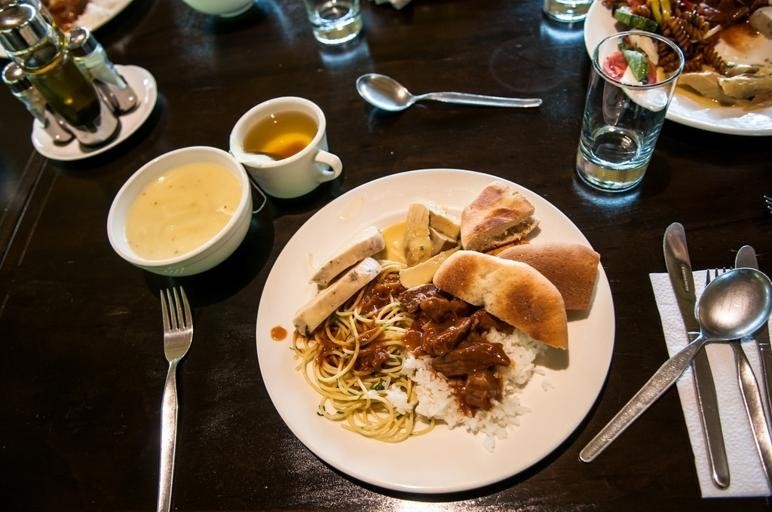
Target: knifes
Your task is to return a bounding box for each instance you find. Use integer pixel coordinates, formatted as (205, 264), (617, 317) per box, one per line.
(662, 221), (729, 490)
(736, 244), (771, 438)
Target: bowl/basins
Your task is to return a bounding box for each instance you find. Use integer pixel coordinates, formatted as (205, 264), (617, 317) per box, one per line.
(106, 146), (254, 280)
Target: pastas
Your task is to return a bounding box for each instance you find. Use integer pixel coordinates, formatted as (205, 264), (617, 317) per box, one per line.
(292, 258), (435, 443)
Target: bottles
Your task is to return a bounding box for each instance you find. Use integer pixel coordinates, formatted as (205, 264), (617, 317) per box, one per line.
(1, 0), (139, 155)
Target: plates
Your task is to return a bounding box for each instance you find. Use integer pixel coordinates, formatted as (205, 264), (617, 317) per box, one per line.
(30, 64), (157, 162)
(255, 167), (619, 498)
(581, 1), (770, 142)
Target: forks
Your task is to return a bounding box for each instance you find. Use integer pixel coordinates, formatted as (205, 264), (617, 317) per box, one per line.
(156, 285), (195, 511)
(703, 265), (771, 492)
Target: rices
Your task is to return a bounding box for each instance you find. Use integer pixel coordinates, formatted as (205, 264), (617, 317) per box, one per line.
(387, 325), (548, 452)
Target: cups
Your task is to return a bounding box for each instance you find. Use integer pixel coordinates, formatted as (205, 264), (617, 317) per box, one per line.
(304, 0), (362, 46)
(576, 34), (686, 201)
(184, 0), (256, 19)
(539, 1), (592, 36)
(230, 96), (344, 201)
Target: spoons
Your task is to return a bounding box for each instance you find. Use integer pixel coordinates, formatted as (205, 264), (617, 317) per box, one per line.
(577, 270), (772, 463)
(354, 73), (542, 115)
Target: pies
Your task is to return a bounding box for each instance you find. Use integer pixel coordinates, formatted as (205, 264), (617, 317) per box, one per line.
(294, 181), (601, 351)
